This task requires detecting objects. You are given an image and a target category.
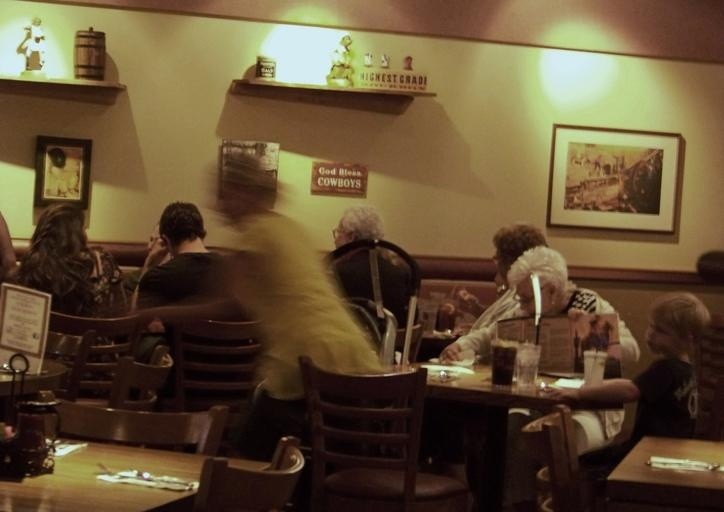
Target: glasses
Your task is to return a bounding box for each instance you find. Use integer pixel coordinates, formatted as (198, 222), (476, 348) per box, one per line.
(332, 228), (345, 239)
(512, 286), (543, 305)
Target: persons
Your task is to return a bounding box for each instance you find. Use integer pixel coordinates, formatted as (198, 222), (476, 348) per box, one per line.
(542, 292), (711, 512)
(1, 151), (412, 511)
(457, 224), (548, 334)
(437, 244), (640, 456)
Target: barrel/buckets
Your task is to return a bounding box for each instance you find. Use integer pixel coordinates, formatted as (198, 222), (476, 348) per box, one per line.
(72, 26), (106, 81)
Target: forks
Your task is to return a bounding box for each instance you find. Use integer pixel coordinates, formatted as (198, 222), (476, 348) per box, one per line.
(91, 460), (190, 489)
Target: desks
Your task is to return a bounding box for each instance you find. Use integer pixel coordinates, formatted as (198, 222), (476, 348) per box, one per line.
(607, 433), (724, 512)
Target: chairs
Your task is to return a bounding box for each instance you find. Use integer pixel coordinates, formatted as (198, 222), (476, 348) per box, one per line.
(679, 314), (723, 441)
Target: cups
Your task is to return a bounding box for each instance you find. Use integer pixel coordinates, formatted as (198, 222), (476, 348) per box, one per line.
(492, 338), (539, 386)
(582, 350), (607, 385)
(421, 300), (454, 333)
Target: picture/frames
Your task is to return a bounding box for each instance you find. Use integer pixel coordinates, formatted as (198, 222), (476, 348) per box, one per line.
(546, 123), (682, 235)
(33, 135), (92, 209)
